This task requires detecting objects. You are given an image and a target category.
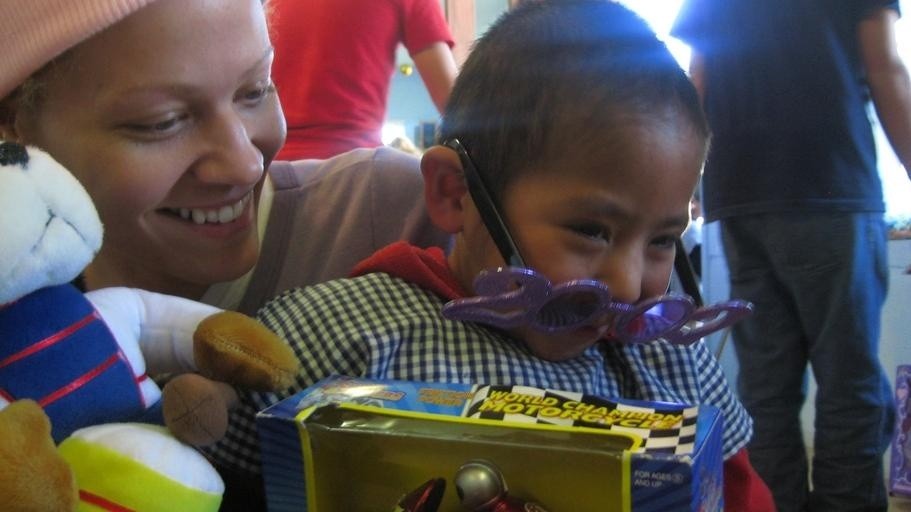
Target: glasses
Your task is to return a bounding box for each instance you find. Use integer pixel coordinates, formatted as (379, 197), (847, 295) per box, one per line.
(435, 131), (756, 347)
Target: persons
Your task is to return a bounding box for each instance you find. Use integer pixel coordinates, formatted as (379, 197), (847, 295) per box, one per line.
(258, 1), (460, 171)
(393, 459), (550, 512)
(0, 1), (460, 512)
(197, 0), (786, 512)
(671, 1), (911, 512)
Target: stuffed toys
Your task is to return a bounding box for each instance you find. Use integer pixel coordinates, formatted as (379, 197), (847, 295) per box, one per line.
(0, 139), (300, 512)
